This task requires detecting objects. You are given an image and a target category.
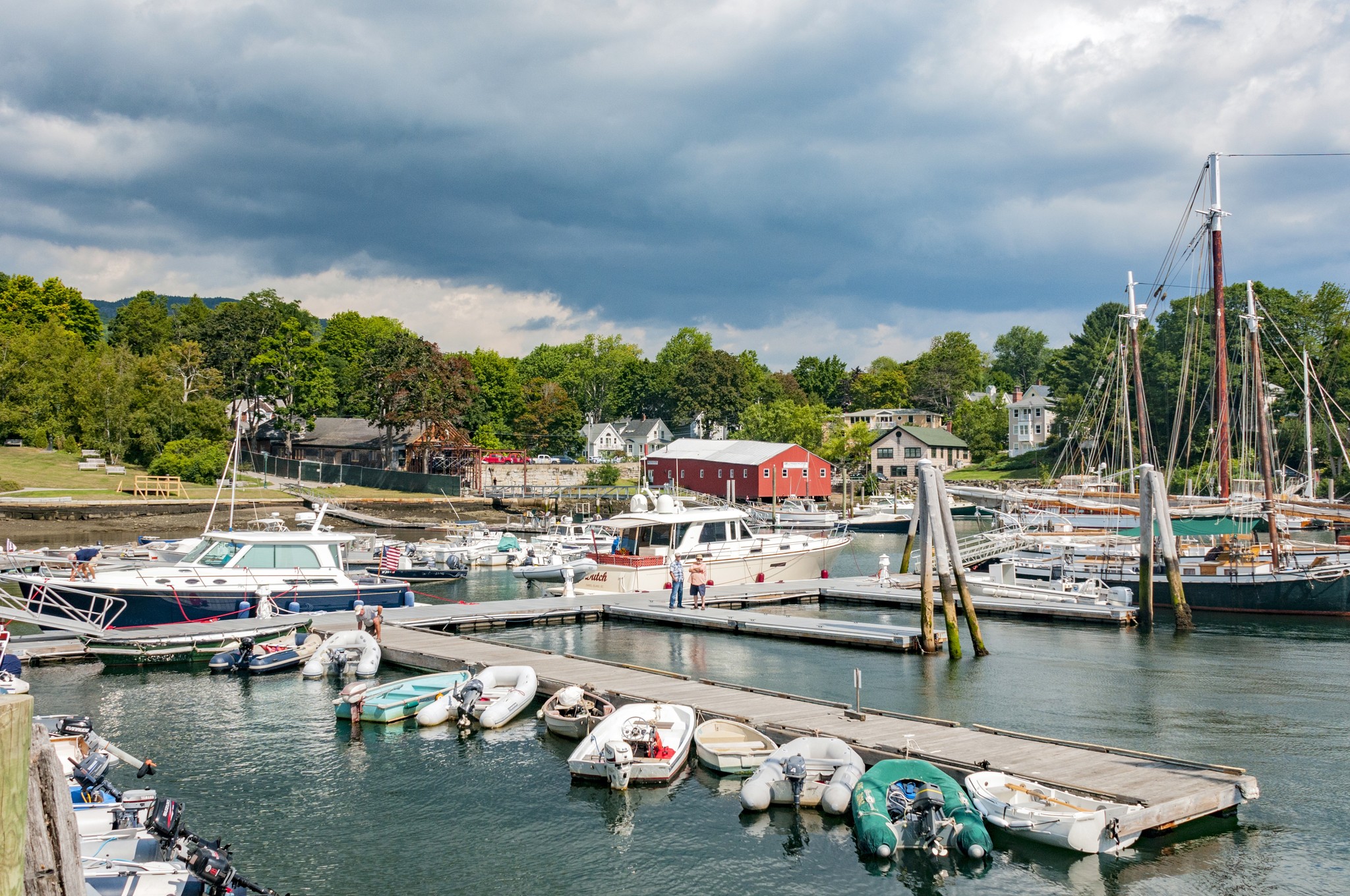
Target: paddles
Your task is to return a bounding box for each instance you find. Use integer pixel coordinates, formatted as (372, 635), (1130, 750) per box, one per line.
(1004, 782), (1096, 812)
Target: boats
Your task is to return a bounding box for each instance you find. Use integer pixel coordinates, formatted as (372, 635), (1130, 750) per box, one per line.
(31, 712), (285, 896)
(852, 757), (994, 864)
(365, 556), (469, 583)
(693, 718), (778, 776)
(738, 736), (866, 815)
(301, 629), (381, 677)
(414, 665), (538, 729)
(965, 771), (1144, 853)
(567, 702), (696, 791)
(332, 669), (472, 724)
(536, 686), (617, 740)
(546, 476), (852, 605)
(0, 447), (919, 588)
(74, 617), (314, 666)
(960, 562), (1134, 607)
(512, 557), (598, 583)
(208, 632), (322, 674)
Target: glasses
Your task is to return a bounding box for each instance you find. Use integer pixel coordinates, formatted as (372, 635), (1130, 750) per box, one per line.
(697, 558), (702, 560)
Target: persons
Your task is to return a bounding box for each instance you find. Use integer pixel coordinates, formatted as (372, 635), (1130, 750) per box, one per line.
(493, 477), (498, 490)
(688, 554), (707, 610)
(67, 549), (102, 582)
(39, 562), (47, 576)
(669, 553), (684, 609)
(239, 636), (255, 655)
(355, 605), (383, 643)
(535, 510), (545, 528)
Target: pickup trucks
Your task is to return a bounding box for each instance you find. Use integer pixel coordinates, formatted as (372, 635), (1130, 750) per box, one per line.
(480, 453), (513, 465)
(504, 454), (533, 464)
(532, 454), (560, 464)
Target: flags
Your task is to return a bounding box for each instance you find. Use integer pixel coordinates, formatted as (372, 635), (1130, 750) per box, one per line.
(381, 545), (400, 570)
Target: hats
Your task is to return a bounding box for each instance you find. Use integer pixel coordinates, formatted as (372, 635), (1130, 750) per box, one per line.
(354, 604), (363, 615)
(696, 554), (703, 559)
(675, 552), (681, 557)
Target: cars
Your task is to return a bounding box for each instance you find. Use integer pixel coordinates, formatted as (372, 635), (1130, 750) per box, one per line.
(586, 456), (600, 464)
(595, 457), (619, 464)
(551, 455), (579, 465)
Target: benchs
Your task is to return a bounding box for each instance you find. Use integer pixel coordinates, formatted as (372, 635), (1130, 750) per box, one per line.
(412, 684), (450, 692)
(384, 689), (429, 700)
(703, 741), (767, 749)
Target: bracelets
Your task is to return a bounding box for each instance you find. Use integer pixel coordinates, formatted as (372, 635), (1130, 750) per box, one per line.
(70, 575), (73, 576)
(73, 575), (76, 577)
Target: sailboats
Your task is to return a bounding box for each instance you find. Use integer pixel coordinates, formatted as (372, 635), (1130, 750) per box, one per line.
(945, 152), (1350, 617)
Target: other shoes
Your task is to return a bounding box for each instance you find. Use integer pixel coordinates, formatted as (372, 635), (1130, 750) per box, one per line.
(677, 606), (686, 609)
(669, 606), (676, 610)
(376, 638), (381, 643)
(701, 606), (705, 610)
(691, 606), (699, 609)
(373, 632), (377, 636)
